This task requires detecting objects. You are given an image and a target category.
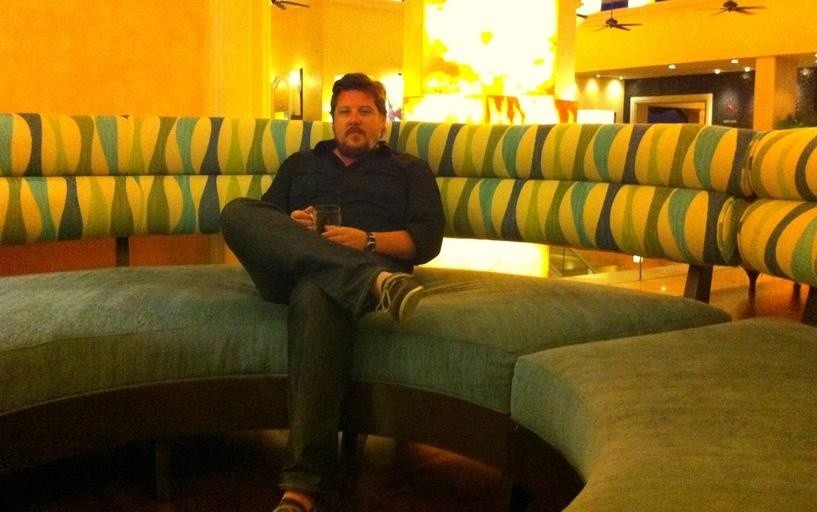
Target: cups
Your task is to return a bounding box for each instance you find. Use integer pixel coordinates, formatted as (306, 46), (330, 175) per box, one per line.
(310, 205), (342, 237)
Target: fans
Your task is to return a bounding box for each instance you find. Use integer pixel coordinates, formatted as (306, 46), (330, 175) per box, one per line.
(706, 1), (765, 20)
(270, 0), (311, 12)
(593, 1), (640, 37)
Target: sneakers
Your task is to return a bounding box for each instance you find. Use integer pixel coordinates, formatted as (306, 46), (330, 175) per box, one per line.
(273, 490), (313, 511)
(377, 274), (424, 320)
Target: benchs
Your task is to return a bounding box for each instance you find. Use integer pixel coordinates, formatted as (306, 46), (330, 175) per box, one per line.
(0, 113), (758, 512)
(509, 126), (817, 511)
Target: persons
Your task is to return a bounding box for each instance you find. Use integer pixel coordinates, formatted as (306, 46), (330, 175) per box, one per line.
(218, 73), (444, 511)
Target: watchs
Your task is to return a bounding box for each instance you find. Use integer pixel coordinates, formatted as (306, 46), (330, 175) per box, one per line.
(364, 230), (375, 251)
